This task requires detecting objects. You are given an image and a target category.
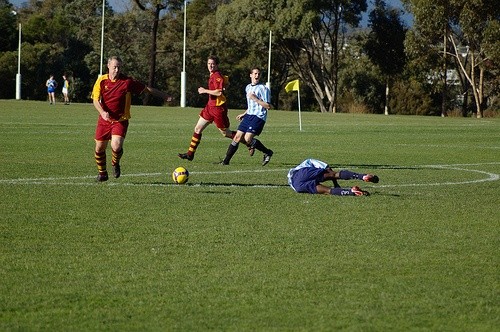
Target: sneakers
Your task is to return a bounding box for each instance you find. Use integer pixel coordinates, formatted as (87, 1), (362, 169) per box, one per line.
(97, 174), (108, 181)
(351, 186), (370, 196)
(112, 163), (120, 178)
(261, 149), (273, 166)
(213, 159), (229, 165)
(247, 142), (255, 156)
(176, 153), (194, 161)
(362, 174), (379, 183)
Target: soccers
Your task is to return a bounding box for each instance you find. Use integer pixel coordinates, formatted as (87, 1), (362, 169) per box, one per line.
(172, 166), (189, 184)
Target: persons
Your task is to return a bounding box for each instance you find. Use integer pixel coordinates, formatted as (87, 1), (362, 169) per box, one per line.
(287, 158), (380, 196)
(62, 74), (70, 105)
(176, 55), (255, 162)
(46, 75), (57, 105)
(90, 53), (132, 182)
(217, 67), (274, 166)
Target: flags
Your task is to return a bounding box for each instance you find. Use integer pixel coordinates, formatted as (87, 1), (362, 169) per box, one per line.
(285, 80), (299, 92)
